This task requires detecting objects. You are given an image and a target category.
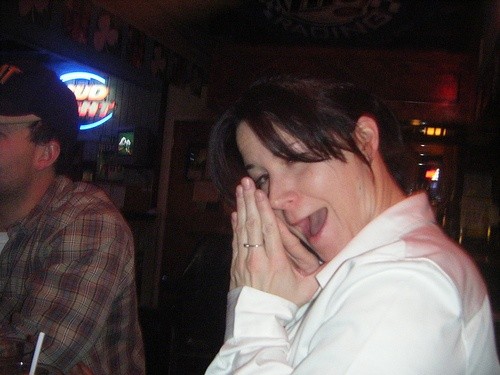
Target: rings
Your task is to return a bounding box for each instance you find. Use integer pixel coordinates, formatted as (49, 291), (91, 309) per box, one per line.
(244, 241), (265, 249)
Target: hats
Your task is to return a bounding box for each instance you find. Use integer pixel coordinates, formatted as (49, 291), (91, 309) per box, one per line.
(1, 54), (80, 161)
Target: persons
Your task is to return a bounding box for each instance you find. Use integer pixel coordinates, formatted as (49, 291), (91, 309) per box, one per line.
(201, 61), (500, 375)
(0, 57), (149, 374)
